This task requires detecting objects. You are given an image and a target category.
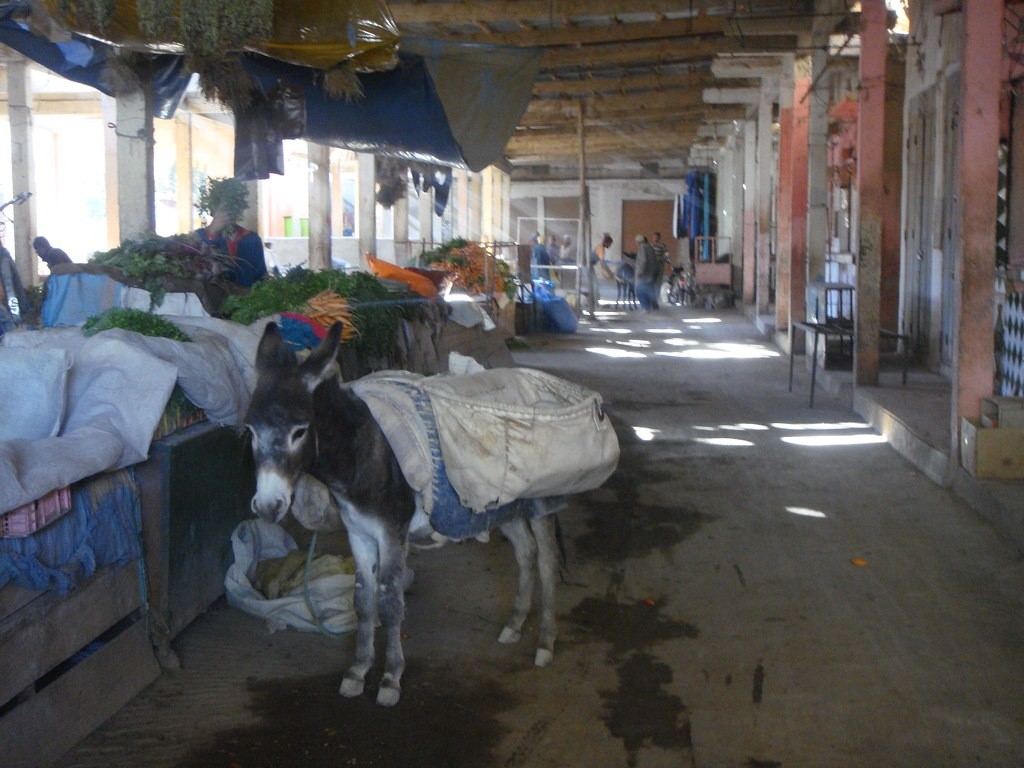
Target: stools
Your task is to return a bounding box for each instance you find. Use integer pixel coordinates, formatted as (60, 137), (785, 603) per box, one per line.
(815, 282), (855, 326)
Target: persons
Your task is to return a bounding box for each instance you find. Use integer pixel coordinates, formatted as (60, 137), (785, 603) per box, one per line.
(0, 236), (74, 342)
(526, 233), (574, 287)
(191, 177), (269, 285)
(623, 232), (668, 312)
(582, 236), (616, 309)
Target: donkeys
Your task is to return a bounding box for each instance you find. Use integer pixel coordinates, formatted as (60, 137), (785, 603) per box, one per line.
(242, 320), (570, 710)
(615, 251), (637, 312)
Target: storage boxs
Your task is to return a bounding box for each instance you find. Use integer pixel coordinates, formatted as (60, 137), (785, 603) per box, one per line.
(960, 416), (1024, 478)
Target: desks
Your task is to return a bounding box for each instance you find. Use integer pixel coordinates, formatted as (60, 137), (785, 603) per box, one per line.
(790, 321), (908, 411)
